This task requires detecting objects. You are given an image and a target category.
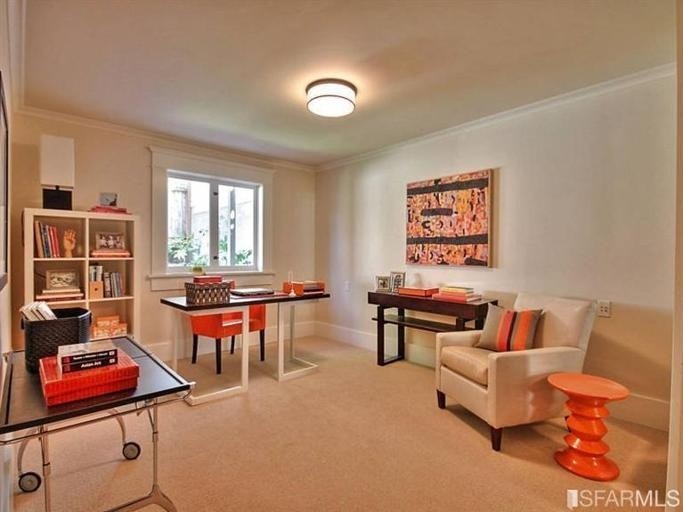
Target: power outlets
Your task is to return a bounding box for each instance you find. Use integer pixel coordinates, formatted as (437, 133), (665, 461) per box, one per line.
(597, 300), (611, 317)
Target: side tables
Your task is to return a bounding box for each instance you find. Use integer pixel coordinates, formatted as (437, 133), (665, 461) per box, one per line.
(548, 372), (631, 481)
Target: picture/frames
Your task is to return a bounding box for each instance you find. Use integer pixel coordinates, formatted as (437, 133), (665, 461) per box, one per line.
(406, 169), (493, 267)
(390, 272), (406, 292)
(46, 269), (79, 290)
(96, 232), (125, 251)
(376, 275), (390, 293)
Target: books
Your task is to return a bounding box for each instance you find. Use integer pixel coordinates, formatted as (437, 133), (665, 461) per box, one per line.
(56, 339), (117, 372)
(34, 221), (60, 258)
(90, 266), (125, 298)
(18, 301), (56, 320)
(432, 287), (481, 303)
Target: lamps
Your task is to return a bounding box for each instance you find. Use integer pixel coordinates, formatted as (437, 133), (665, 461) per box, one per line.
(304, 78), (357, 118)
(38, 134), (76, 210)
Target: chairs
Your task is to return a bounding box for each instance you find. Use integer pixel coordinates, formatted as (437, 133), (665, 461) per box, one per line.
(435, 292), (596, 451)
(191, 277), (266, 374)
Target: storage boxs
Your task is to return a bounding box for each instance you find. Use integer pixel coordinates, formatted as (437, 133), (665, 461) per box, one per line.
(185, 282), (233, 304)
(35, 352), (139, 407)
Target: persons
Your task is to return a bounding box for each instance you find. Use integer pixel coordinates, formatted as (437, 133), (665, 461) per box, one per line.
(63, 228), (76, 258)
(100, 235), (122, 248)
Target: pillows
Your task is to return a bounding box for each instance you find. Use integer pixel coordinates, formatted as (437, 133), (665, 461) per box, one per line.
(474, 302), (542, 352)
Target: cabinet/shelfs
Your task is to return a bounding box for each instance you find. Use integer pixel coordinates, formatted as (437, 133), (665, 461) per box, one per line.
(23, 206), (139, 341)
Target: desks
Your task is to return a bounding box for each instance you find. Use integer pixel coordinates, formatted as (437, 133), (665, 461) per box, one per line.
(368, 288), (498, 366)
(159, 289), (330, 405)
(0, 336), (196, 512)
